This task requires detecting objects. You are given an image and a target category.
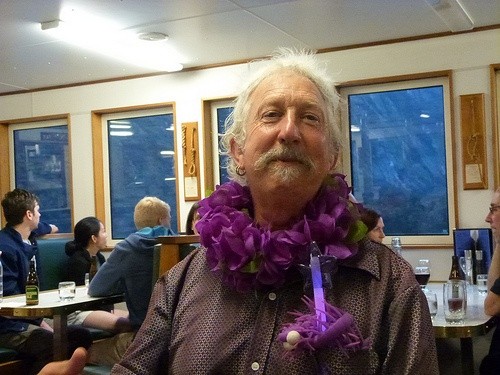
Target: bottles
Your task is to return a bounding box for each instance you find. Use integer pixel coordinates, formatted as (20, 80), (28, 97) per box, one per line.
(447, 255), (467, 310)
(24, 261), (39, 305)
(89, 255), (98, 285)
(390, 236), (402, 256)
(0, 259), (4, 303)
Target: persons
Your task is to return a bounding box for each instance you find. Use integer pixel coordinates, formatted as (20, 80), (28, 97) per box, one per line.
(0, 188), (93, 375)
(65, 217), (131, 333)
(479, 187), (500, 375)
(37, 47), (440, 375)
(359, 208), (385, 243)
(186, 202), (201, 247)
(86, 196), (175, 365)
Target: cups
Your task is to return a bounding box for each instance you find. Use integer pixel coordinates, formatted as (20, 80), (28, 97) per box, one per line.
(58, 281), (76, 301)
(476, 274), (487, 292)
(442, 279), (468, 325)
(84, 273), (89, 288)
(420, 285), (437, 320)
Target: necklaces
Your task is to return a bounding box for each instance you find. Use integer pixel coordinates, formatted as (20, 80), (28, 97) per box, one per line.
(195, 173), (371, 300)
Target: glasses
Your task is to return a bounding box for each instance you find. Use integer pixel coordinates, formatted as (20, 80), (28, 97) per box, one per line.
(489, 206), (500, 214)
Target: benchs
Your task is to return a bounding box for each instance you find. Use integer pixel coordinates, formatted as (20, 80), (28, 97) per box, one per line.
(0, 234), (201, 375)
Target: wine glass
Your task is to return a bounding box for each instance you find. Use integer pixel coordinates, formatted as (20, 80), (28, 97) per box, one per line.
(413, 259), (429, 291)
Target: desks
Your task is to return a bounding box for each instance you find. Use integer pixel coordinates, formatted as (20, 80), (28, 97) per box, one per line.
(418, 283), (496, 375)
(0, 283), (126, 362)
